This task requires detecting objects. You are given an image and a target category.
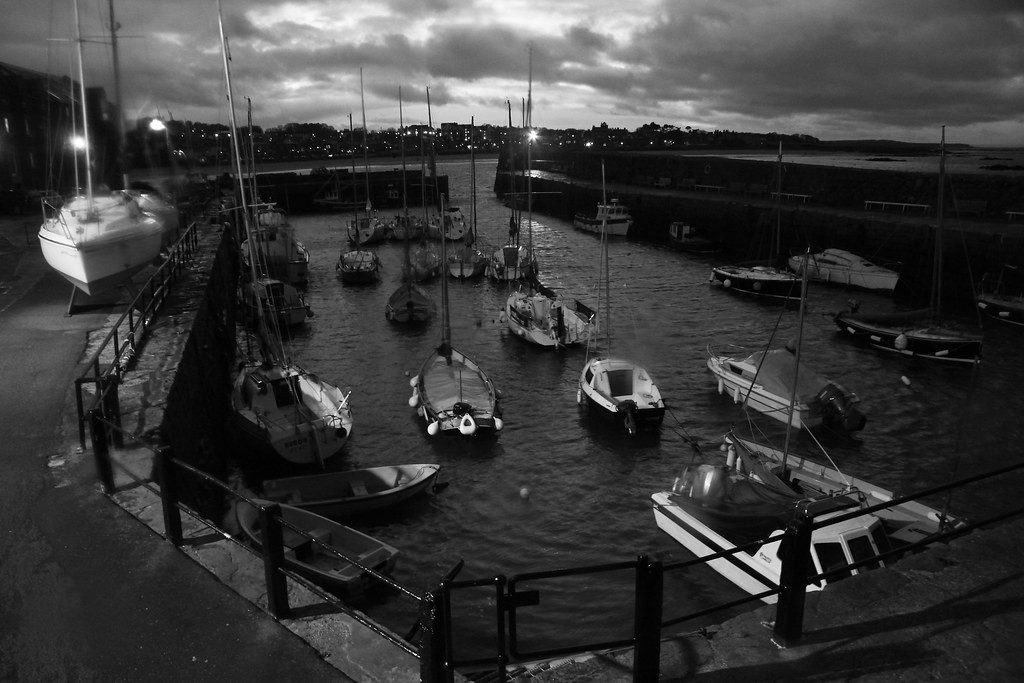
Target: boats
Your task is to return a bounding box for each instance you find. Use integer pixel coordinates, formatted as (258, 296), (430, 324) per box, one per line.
(787, 248), (901, 292)
(649, 435), (897, 605)
(235, 498), (401, 592)
(573, 198), (634, 237)
(259, 463), (442, 513)
(705, 347), (867, 434)
(977, 264), (1024, 326)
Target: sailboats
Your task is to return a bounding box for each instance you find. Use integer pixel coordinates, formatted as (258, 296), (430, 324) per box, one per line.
(577, 162), (664, 436)
(385, 85), (437, 325)
(228, 96), (311, 328)
(346, 67), (538, 285)
(708, 139), (809, 302)
(226, 36), (354, 470)
(506, 49), (599, 349)
(829, 124), (986, 366)
(335, 113), (382, 281)
(719, 248), (981, 550)
(37, 1), (183, 298)
(408, 192), (504, 438)
